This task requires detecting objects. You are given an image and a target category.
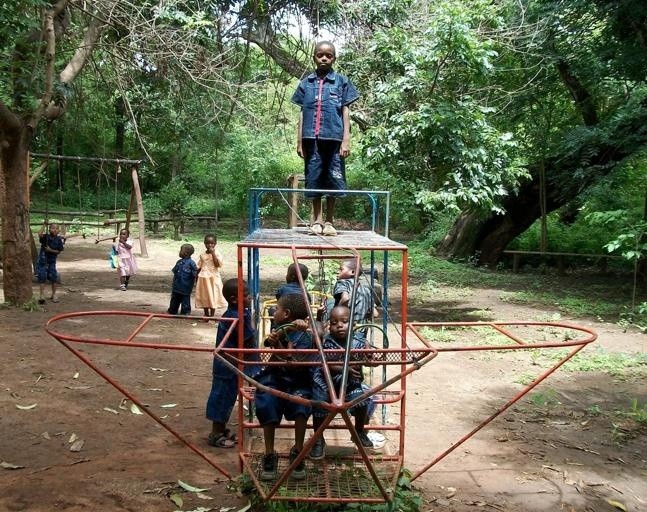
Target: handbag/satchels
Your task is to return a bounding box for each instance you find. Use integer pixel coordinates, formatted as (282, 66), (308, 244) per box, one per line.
(110, 255), (118, 268)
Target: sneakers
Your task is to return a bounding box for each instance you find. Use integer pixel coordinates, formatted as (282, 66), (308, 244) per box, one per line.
(50, 295), (59, 302)
(120, 284), (126, 290)
(260, 453), (279, 480)
(39, 297), (45, 304)
(288, 445), (306, 479)
(309, 438), (327, 460)
(308, 221), (323, 234)
(351, 432), (374, 450)
(323, 222), (337, 235)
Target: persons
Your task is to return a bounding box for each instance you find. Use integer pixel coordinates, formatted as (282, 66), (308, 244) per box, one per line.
(317, 258), (379, 337)
(196, 234), (228, 322)
(266, 262), (312, 333)
(167, 243), (197, 315)
(252, 289), (319, 482)
(34, 220), (64, 303)
(290, 40), (360, 237)
(205, 277), (255, 447)
(305, 304), (384, 461)
(112, 228), (138, 290)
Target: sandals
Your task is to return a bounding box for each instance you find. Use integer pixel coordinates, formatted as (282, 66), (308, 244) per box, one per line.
(208, 429), (238, 448)
